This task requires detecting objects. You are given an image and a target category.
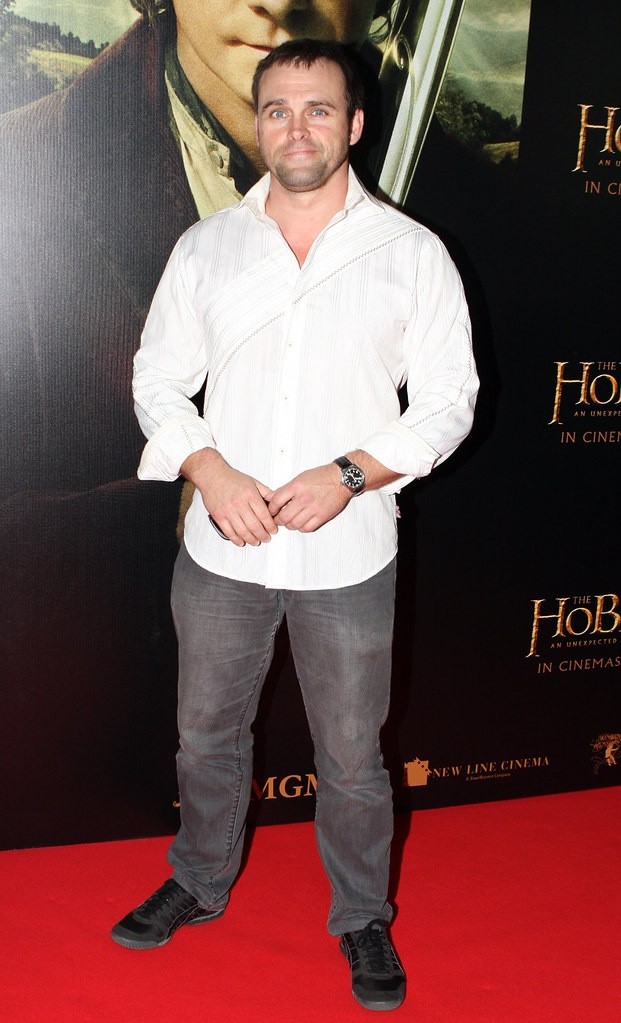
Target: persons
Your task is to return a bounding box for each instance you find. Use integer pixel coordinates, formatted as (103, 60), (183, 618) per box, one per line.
(0, 1), (451, 535)
(107, 37), (485, 1013)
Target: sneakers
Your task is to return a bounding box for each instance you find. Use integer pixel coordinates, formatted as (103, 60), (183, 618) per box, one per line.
(340, 929), (407, 1011)
(110, 877), (228, 948)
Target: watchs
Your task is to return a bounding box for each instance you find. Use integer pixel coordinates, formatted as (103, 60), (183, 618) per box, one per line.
(332, 455), (366, 499)
